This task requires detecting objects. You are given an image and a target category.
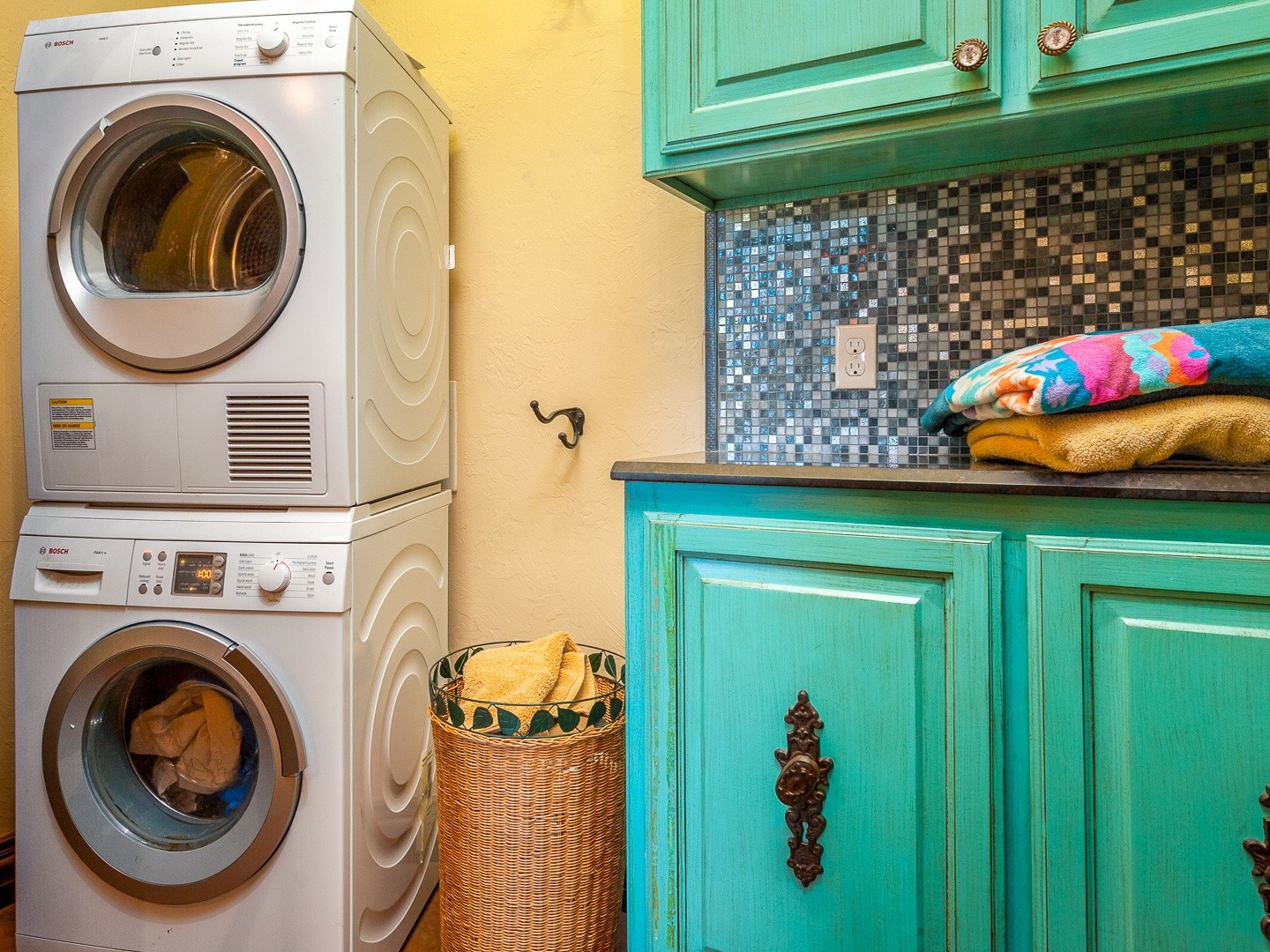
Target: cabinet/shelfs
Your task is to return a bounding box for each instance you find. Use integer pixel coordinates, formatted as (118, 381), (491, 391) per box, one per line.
(611, 453), (1270, 951)
(641, 1), (1269, 212)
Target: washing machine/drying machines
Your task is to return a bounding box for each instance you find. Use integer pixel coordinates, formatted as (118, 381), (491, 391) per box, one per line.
(12, 0), (452, 514)
(4, 487), (442, 952)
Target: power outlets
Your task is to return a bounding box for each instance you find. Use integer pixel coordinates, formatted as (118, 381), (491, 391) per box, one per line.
(834, 324), (877, 390)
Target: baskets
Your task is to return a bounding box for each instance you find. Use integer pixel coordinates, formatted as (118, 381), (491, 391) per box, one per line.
(420, 637), (627, 952)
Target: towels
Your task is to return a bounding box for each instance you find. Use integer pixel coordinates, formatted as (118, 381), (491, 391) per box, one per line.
(917, 315), (1270, 438)
(966, 395), (1270, 473)
(448, 631), (606, 740)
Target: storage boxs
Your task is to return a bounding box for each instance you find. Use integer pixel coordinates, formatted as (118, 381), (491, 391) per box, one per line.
(428, 640), (626, 952)
